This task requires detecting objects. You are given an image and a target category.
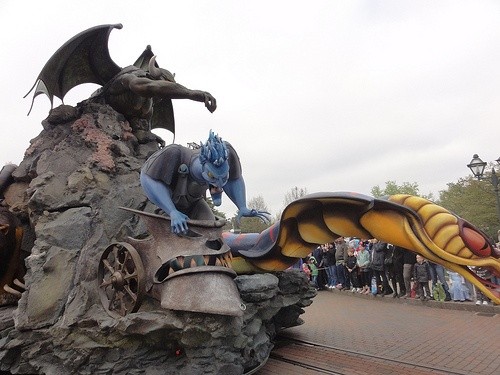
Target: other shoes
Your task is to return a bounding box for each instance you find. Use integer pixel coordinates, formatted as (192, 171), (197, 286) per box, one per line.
(393, 292), (398, 298)
(329, 286), (332, 289)
(365, 289), (371, 295)
(360, 289), (366, 294)
(332, 285), (336, 288)
(357, 287), (362, 293)
(476, 301), (481, 305)
(483, 300), (488, 305)
(426, 295), (431, 300)
(352, 288), (357, 292)
(399, 293), (411, 299)
(397, 292), (404, 298)
(420, 295), (424, 300)
(337, 284), (350, 290)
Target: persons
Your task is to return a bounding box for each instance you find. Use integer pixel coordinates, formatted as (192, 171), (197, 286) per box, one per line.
(490, 241), (500, 286)
(412, 253), (434, 301)
(473, 265), (491, 305)
(425, 257), (452, 303)
(288, 235), (406, 298)
(401, 247), (419, 299)
(449, 269), (471, 301)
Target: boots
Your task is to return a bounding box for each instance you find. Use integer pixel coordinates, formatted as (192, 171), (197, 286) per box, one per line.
(444, 292), (451, 301)
